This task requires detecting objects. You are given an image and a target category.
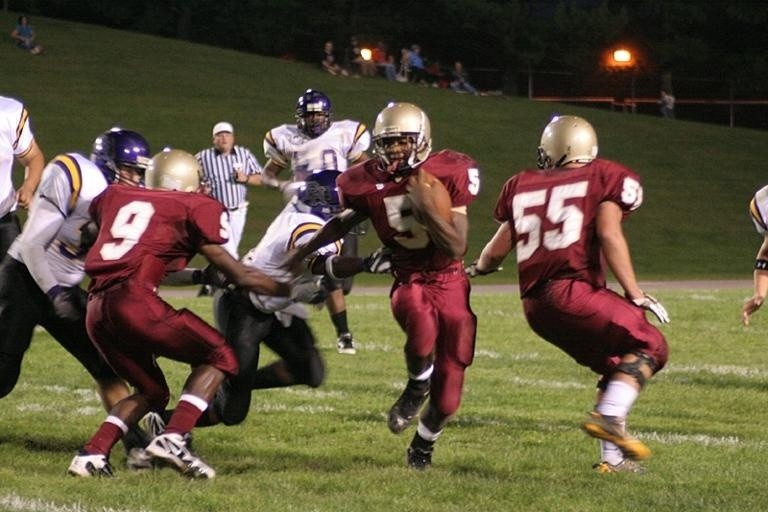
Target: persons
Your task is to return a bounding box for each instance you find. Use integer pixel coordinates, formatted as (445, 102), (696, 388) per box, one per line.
(273, 101), (482, 469)
(0, 95), (46, 266)
(741, 184), (768, 328)
(157, 169), (395, 429)
(66, 147), (328, 482)
(462, 113), (670, 476)
(0, 125), (160, 471)
(319, 34), (480, 98)
(261, 88), (372, 355)
(8, 13), (46, 57)
(192, 120), (263, 297)
(657, 90), (676, 120)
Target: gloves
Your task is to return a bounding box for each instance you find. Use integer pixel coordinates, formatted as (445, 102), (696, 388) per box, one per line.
(464, 259), (504, 277)
(364, 245), (397, 276)
(47, 285), (83, 325)
(628, 289), (671, 325)
(285, 280), (326, 305)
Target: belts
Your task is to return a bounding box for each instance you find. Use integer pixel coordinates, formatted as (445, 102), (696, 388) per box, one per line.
(224, 204), (247, 212)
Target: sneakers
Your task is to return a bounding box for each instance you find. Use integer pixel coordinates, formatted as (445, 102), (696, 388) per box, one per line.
(65, 452), (117, 482)
(125, 446), (152, 473)
(335, 332), (356, 355)
(579, 409), (651, 462)
(590, 457), (644, 476)
(143, 431), (217, 482)
(404, 444), (434, 475)
(141, 410), (167, 438)
(386, 378), (433, 435)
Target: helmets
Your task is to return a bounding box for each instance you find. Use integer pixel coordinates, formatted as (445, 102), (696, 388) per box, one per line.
(373, 102), (433, 177)
(89, 126), (149, 191)
(143, 147), (199, 197)
(294, 89), (332, 136)
(534, 113), (599, 169)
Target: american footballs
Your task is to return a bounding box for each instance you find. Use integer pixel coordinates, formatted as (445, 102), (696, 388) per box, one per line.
(411, 172), (454, 227)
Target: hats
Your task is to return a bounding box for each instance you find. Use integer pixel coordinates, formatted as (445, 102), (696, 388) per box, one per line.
(210, 121), (235, 137)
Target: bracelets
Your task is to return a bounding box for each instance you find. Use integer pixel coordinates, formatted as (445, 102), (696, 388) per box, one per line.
(244, 175), (250, 185)
